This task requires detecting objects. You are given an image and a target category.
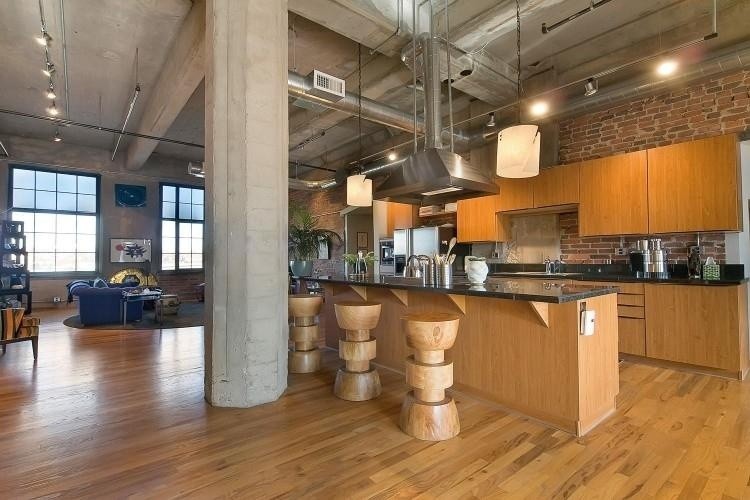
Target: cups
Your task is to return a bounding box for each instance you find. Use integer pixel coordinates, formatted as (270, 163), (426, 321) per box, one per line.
(423, 263), (455, 285)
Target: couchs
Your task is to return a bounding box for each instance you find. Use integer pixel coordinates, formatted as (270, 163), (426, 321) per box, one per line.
(61, 273), (144, 328)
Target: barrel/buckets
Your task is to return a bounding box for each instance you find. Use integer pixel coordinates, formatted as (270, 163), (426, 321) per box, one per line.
(155, 295), (180, 315)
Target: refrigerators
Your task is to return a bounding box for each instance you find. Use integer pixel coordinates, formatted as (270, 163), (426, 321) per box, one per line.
(393, 226), (470, 277)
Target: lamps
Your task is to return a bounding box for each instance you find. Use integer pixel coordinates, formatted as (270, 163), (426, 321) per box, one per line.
(483, 71), (601, 128)
(36, 24), (65, 144)
(341, 41), (378, 210)
(490, 0), (544, 181)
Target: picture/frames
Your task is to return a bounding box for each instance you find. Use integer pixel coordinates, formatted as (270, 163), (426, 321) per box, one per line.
(108, 236), (153, 265)
(357, 248), (369, 256)
(355, 230), (369, 249)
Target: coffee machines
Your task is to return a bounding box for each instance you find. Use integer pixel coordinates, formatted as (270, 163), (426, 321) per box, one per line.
(628, 239), (670, 279)
(380, 238), (394, 274)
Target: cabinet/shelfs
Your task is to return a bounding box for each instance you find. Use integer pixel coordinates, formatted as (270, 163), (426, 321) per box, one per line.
(0, 219), (36, 316)
(456, 175), (513, 248)
(578, 131), (746, 241)
(493, 162), (580, 219)
(571, 278), (645, 364)
(643, 284), (749, 381)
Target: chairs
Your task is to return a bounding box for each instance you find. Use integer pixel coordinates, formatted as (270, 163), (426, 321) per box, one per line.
(0, 305), (40, 363)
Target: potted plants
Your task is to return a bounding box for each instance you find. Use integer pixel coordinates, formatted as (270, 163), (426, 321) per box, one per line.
(286, 200), (347, 279)
(341, 250), (379, 274)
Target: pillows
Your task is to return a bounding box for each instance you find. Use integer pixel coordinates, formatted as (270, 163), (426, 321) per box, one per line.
(91, 276), (111, 289)
(87, 279), (94, 289)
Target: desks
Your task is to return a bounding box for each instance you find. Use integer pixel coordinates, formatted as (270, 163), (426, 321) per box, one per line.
(117, 291), (166, 329)
(290, 275), (624, 440)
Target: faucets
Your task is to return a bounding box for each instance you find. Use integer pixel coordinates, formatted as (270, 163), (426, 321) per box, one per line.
(544, 256), (552, 274)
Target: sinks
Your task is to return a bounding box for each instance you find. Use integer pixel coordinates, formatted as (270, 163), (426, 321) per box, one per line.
(492, 270), (584, 277)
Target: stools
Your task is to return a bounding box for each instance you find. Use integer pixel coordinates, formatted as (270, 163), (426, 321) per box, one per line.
(153, 292), (183, 316)
(287, 289), (329, 380)
(394, 309), (466, 442)
(330, 298), (388, 406)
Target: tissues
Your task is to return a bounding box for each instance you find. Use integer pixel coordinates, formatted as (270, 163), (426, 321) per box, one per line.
(701, 257), (721, 280)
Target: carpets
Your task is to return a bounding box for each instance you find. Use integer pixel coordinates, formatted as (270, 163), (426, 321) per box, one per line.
(62, 298), (208, 331)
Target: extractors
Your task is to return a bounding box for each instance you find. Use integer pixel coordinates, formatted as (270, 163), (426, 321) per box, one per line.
(371, 149), (500, 208)
